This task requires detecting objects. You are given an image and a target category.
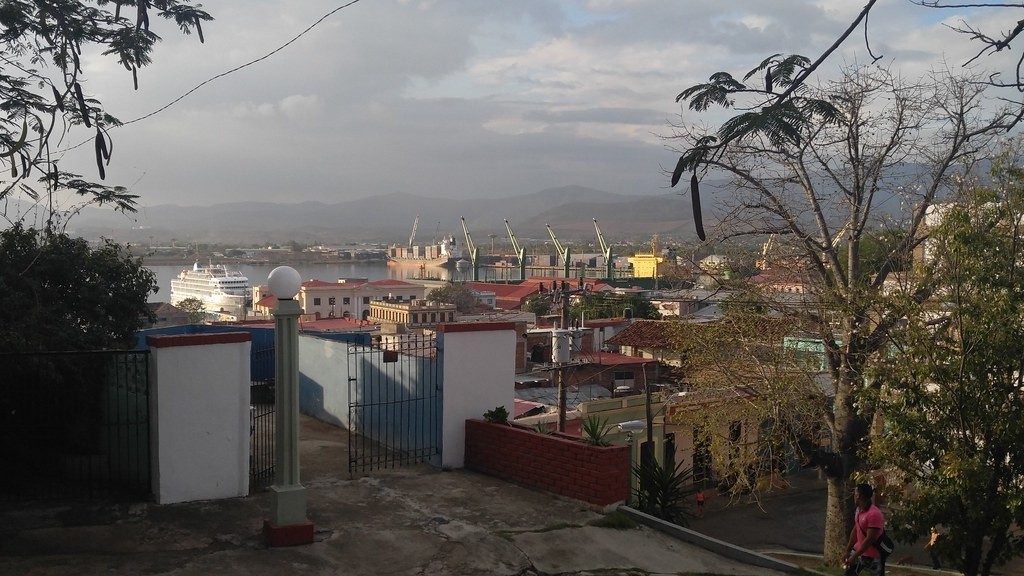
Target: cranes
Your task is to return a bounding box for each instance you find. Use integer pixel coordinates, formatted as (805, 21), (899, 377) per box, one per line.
(592, 218), (614, 278)
(409, 215), (421, 247)
(460, 215), (483, 283)
(502, 217), (532, 285)
(546, 223), (572, 278)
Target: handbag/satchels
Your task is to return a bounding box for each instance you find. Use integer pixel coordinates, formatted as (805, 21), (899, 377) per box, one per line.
(874, 533), (894, 556)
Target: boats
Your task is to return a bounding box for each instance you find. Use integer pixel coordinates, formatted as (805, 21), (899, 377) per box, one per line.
(170, 258), (254, 310)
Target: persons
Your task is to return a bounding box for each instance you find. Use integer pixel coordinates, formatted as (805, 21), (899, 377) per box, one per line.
(695, 487), (706, 517)
(923, 525), (944, 569)
(842, 484), (885, 576)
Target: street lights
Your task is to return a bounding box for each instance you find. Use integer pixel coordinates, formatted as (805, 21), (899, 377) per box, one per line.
(645, 391), (689, 444)
(263, 267), (318, 547)
